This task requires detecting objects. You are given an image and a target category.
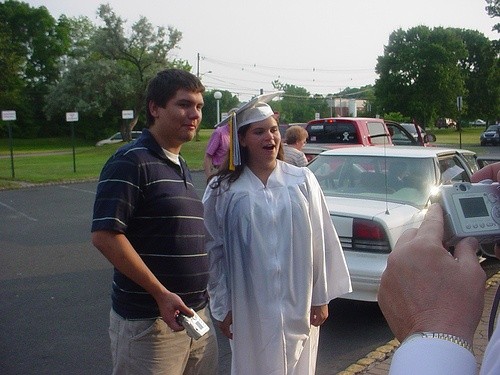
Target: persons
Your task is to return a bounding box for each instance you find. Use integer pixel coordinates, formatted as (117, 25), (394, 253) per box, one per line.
(204, 108), (231, 178)
(200, 90), (353, 375)
(378, 162), (500, 375)
(91, 70), (218, 375)
(278, 126), (308, 168)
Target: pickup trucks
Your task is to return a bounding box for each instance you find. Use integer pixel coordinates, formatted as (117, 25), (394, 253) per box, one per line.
(469, 119), (487, 126)
(281, 116), (437, 163)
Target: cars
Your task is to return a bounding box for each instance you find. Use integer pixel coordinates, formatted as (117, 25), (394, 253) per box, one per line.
(394, 122), (428, 142)
(96, 130), (142, 146)
(437, 118), (457, 129)
(305, 143), (499, 303)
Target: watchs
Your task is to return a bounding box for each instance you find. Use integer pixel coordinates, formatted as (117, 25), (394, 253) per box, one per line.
(396, 331), (474, 354)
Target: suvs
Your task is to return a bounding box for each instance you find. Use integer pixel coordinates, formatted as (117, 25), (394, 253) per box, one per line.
(480, 124), (500, 146)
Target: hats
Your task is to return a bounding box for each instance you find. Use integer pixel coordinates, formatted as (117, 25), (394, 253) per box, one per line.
(214, 90), (286, 170)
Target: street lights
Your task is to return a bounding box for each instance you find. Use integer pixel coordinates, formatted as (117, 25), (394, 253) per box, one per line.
(214, 91), (223, 124)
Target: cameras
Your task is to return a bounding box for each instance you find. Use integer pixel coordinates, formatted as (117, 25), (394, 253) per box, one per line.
(177, 307), (210, 341)
(428, 180), (500, 247)
